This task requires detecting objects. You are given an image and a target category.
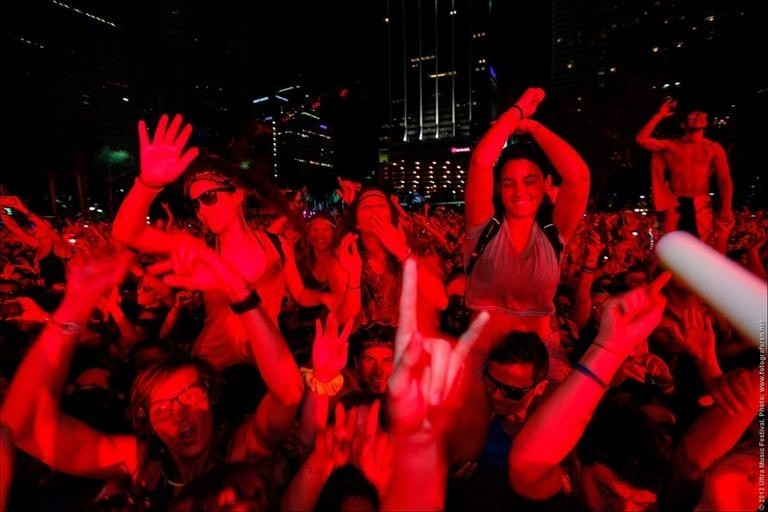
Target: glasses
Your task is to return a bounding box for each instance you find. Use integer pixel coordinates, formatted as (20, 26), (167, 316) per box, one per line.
(487, 375), (534, 399)
(145, 383), (204, 420)
(188, 186), (236, 210)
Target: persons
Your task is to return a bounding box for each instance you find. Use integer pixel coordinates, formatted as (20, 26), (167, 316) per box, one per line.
(0, 86), (768, 512)
